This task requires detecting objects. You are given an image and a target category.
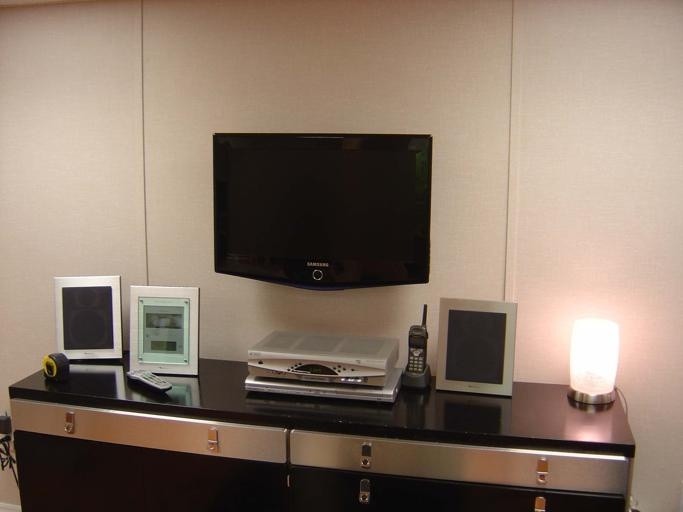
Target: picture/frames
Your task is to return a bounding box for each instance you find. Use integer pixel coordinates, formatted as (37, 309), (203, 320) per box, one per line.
(436, 297), (518, 398)
(130, 286), (200, 377)
(55, 275), (123, 359)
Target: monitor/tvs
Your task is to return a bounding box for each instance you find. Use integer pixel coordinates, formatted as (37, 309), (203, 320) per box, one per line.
(211, 131), (429, 290)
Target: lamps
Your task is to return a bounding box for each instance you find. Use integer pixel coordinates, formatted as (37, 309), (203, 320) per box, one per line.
(567, 316), (618, 405)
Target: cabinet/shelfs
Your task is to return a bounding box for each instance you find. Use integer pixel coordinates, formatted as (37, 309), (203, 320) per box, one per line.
(10, 384), (289, 512)
(287, 429), (636, 511)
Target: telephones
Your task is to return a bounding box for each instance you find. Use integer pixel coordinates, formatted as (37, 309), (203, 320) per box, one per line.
(402, 304), (431, 389)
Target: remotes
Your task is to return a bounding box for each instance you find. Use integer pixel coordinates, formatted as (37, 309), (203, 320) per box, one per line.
(125, 369), (172, 392)
